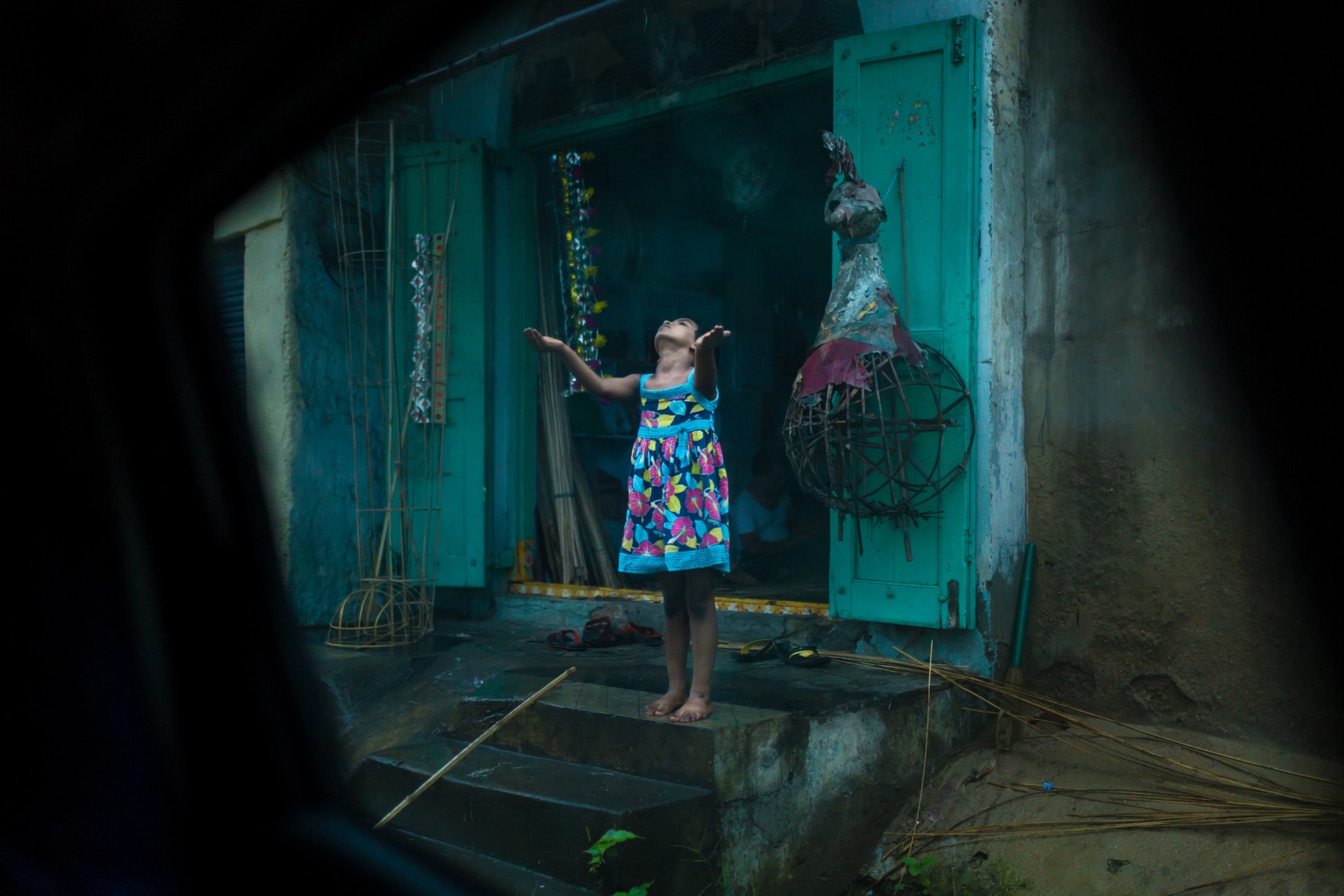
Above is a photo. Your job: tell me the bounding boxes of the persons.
[525,316,733,723]
